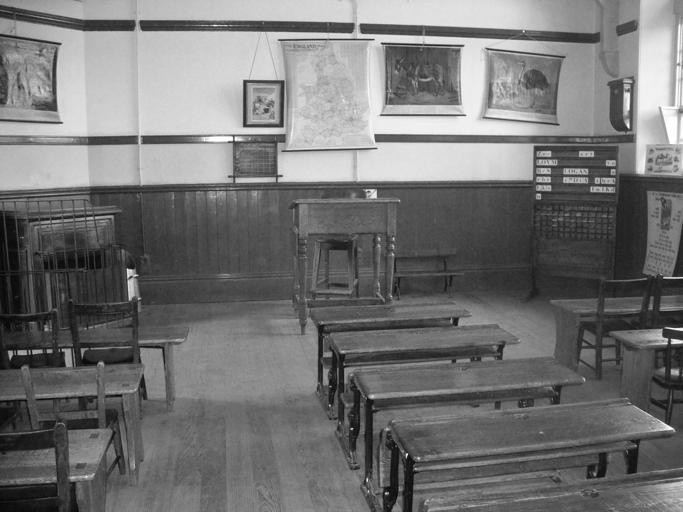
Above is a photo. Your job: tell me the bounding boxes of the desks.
[0,326,189,411]
[287,197,401,335]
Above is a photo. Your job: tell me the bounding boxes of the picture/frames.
[242,79,284,128]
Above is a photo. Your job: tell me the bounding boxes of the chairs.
[307,188,367,298]
[575,273,683,379]
[393,247,464,300]
[0,296,148,503]
